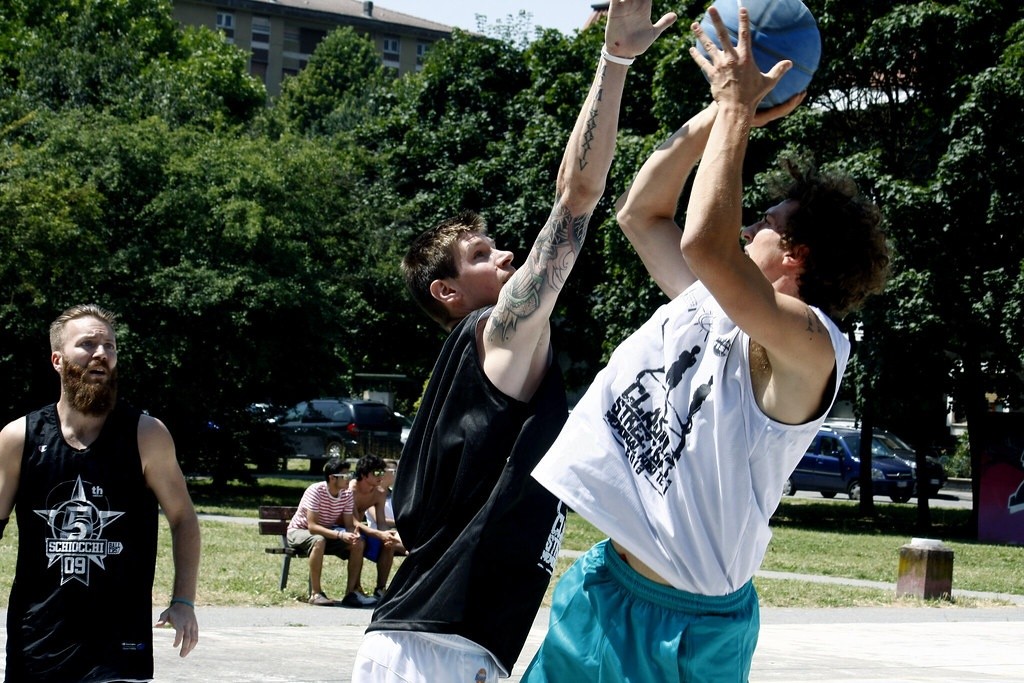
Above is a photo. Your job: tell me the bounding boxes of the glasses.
[332,472,355,481]
[367,470,386,477]
[386,468,397,474]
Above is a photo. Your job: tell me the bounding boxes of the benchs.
[258,505,408,594]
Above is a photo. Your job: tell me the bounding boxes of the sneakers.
[308,591,336,605]
[374,586,387,600]
[341,587,378,607]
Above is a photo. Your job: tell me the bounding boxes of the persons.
[364,457,415,555]
[345,455,401,603]
[272,457,377,606]
[2,302,201,683]
[507,5,886,683]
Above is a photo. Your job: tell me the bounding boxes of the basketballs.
[696,0,819,110]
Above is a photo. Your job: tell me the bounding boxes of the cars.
[860,430,948,497]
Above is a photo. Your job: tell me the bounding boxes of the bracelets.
[336,530,339,539]
[170,597,196,608]
[340,0,681,683]
[340,530,344,539]
[599,45,637,66]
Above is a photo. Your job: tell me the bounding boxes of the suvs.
[268,395,403,459]
[783,423,917,504]
